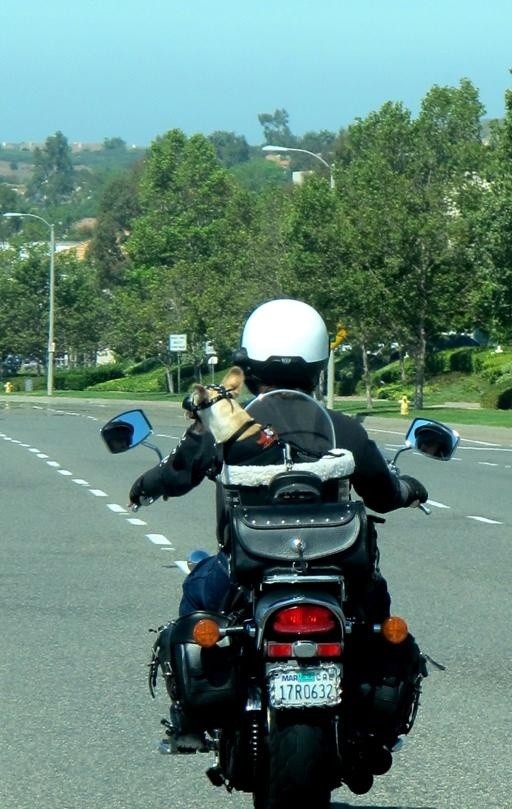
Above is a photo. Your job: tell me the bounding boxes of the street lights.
[262,144,335,410]
[3,211,56,398]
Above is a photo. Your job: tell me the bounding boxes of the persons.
[412,424,452,457]
[102,421,133,451]
[128,296,419,751]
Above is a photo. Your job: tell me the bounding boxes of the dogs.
[181,366,313,467]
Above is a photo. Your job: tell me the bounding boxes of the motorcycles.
[103,385,462,809]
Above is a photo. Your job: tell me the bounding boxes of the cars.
[391,333,478,361]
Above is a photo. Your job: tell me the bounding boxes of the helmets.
[241,299,330,370]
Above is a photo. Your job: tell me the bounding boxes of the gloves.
[397,475,428,506]
[129,476,148,506]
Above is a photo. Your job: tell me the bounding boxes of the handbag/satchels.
[230,502,368,585]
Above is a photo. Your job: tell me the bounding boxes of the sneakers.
[170,705,205,749]
